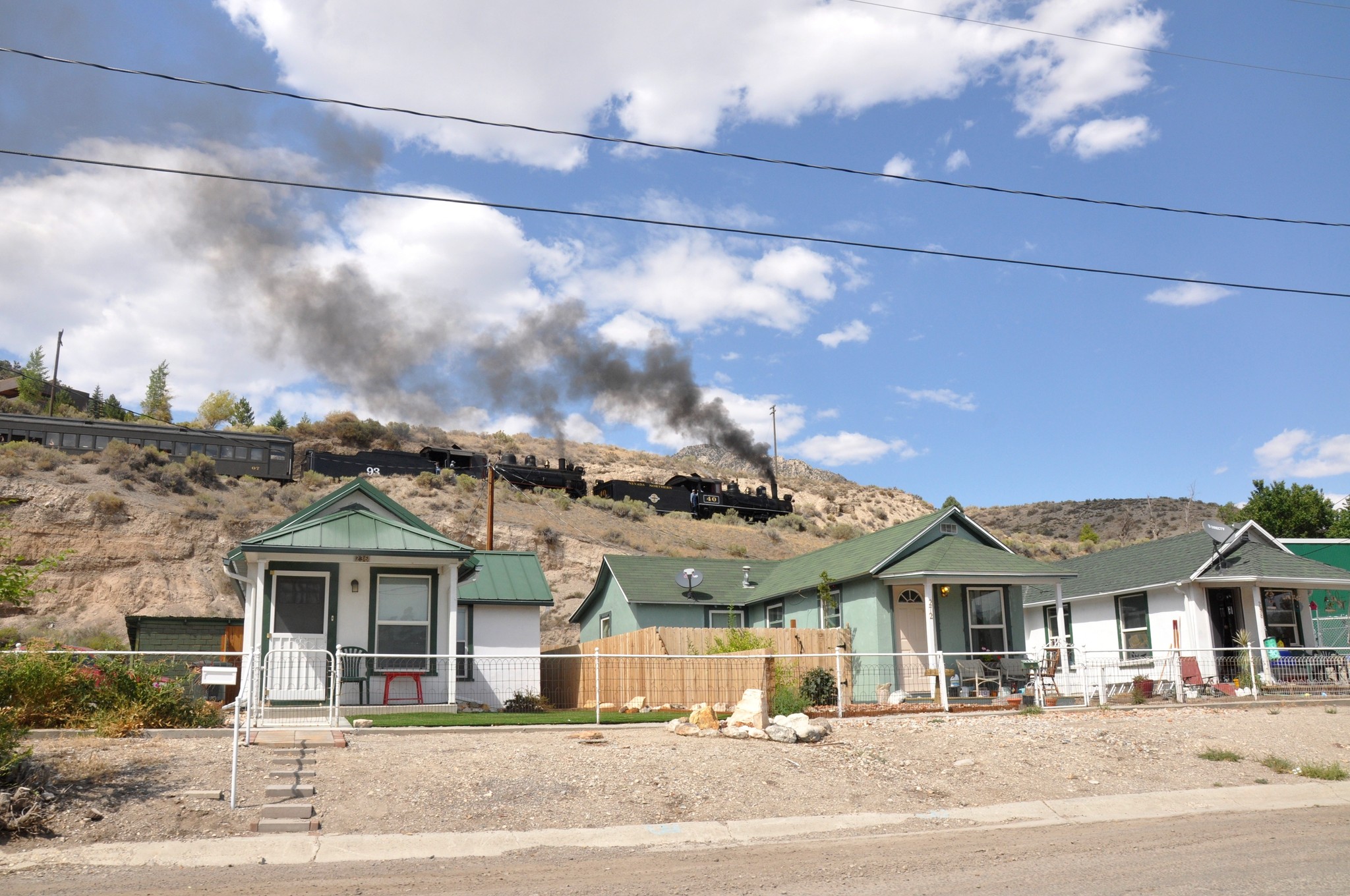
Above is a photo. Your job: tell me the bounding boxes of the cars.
[0,642,181,712]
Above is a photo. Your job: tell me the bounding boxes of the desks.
[382,672,426,706]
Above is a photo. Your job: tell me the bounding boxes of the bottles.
[1277,639,1284,647]
[950,673,960,687]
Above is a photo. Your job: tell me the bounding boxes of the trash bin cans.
[192,660,233,703]
[1264,636,1281,660]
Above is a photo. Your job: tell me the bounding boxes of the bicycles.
[1167,675,1223,697]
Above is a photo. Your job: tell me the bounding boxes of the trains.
[0,413,796,525]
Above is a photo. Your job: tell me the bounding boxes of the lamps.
[938,583,951,598]
[351,579,359,593]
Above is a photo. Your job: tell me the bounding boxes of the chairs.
[1026,648,1062,697]
[1312,649,1350,685]
[999,657,1036,694]
[1269,651,1297,682]
[1288,650,1328,685]
[334,646,374,705]
[955,659,1002,697]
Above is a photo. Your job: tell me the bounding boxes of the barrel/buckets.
[997,682,1012,697]
